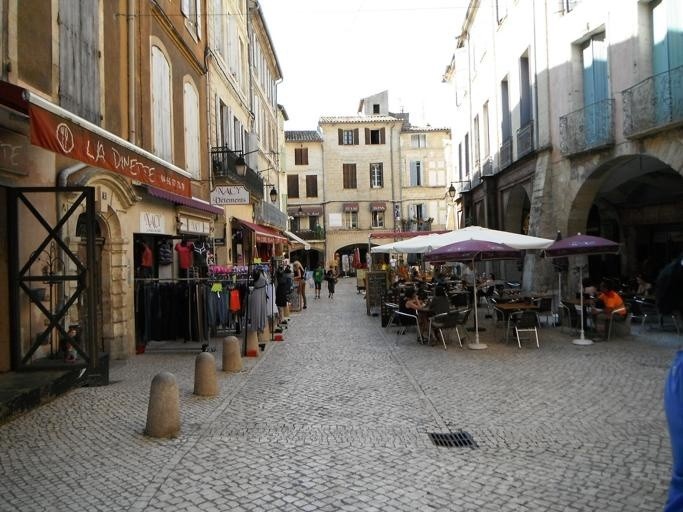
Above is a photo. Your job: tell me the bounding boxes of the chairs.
[384,263,683,350]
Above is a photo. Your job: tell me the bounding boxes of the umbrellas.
[371,232,440,253]
[541,230,623,330]
[351,248,362,269]
[389,224,554,340]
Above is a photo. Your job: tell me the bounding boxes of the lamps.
[449,180,473,198]
[234,150,247,178]
[268,183,279,206]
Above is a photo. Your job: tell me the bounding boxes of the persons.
[398,256,404,264]
[426,284,450,347]
[476,272,494,308]
[389,255,396,268]
[461,264,480,306]
[293,260,306,309]
[411,271,426,282]
[632,273,652,295]
[252,264,266,332]
[272,265,288,323]
[310,263,321,299]
[589,278,626,340]
[325,265,336,298]
[398,287,427,342]
[264,273,283,333]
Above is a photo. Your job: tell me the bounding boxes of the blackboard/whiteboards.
[365,271,388,306]
